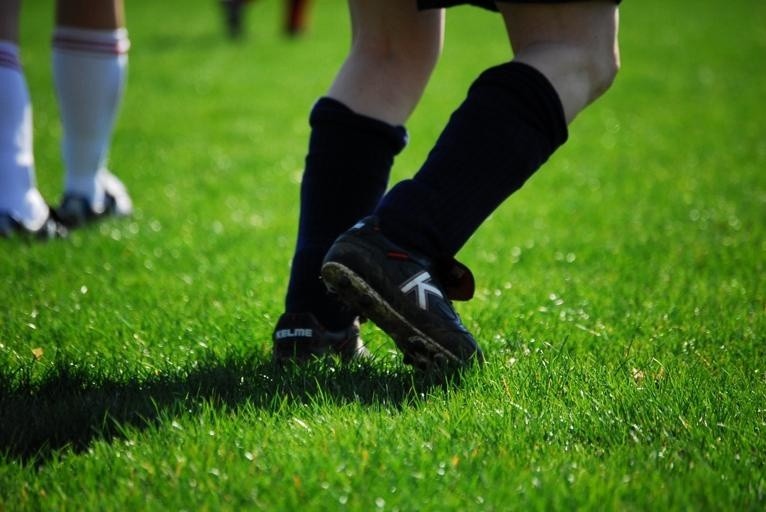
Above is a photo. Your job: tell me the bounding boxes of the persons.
[219,0,317,43]
[269,1,626,393]
[0,0,135,249]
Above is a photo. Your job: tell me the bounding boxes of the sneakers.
[322,214,485,384]
[1,205,77,237]
[272,311,371,361]
[60,185,115,226]
